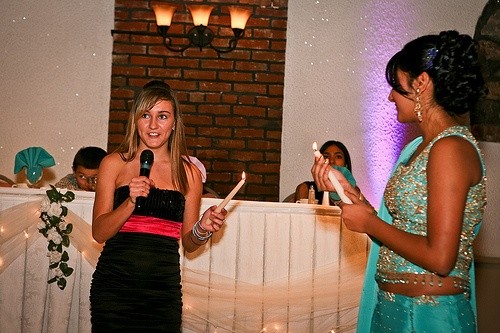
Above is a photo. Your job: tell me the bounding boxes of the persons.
[296,139,361,204]
[311,29,487,333]
[89,80,227,333]
[55,146,105,191]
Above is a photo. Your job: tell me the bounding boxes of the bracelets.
[190,214,212,246]
[359,193,364,202]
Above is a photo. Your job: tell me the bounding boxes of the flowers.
[37,184,74,290]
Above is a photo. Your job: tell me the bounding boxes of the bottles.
[308,185,316,204]
[322,191,329,206]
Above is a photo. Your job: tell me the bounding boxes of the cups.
[296,199,319,204]
[12,183,27,188]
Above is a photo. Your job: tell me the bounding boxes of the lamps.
[149,2,253,52]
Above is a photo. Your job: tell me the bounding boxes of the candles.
[206,171,246,225]
[312,142,354,204]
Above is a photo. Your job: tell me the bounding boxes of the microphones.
[135,150,154,207]
